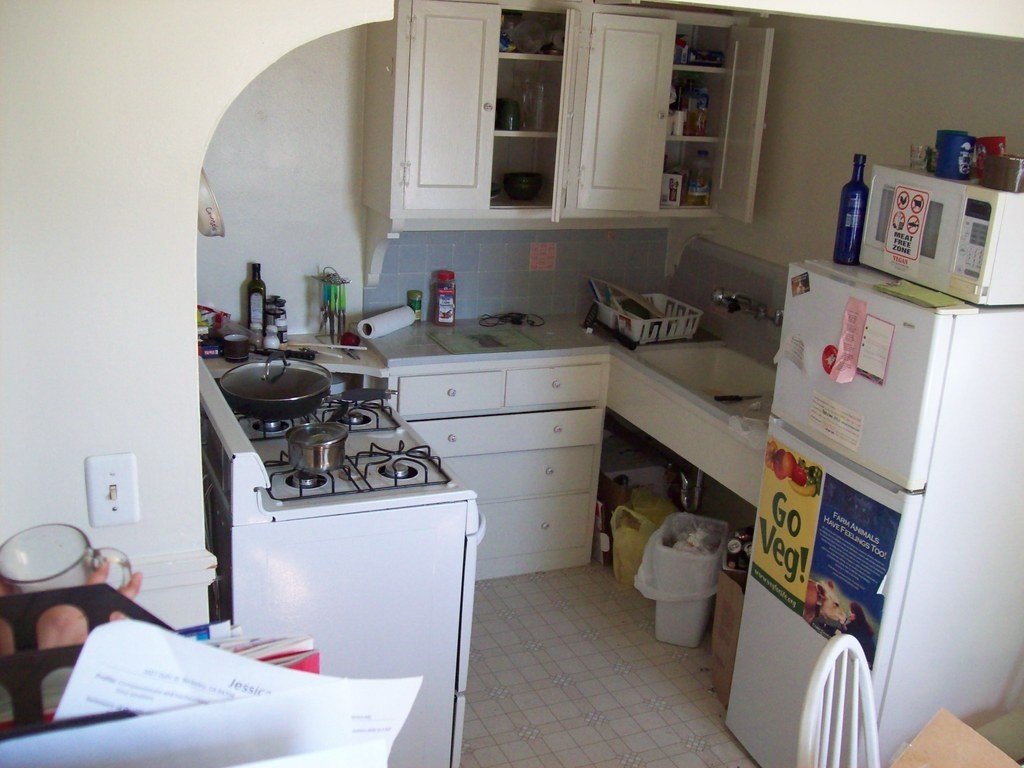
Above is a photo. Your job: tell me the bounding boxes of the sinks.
[634,347,776,407]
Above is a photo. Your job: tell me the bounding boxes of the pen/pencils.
[299,348,342,358]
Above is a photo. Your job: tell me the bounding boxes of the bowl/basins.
[504,172,544,200]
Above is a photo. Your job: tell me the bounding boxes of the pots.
[220,351,333,420]
[285,401,349,473]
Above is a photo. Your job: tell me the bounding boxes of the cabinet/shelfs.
[362,0,779,287]
[387,354,611,581]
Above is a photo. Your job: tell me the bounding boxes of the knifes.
[322,282,346,345]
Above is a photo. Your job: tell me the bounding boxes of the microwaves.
[858,164,1024,305]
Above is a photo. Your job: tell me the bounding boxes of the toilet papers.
[356,305,416,339]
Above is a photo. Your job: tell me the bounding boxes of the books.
[0,618,320,731]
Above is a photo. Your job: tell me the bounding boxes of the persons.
[841,602,877,671]
[0,561,143,655]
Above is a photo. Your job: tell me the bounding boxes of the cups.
[223,334,249,363]
[494,98,522,131]
[522,81,558,131]
[980,155,1024,193]
[934,134,976,180]
[976,136,1006,178]
[910,145,928,169]
[0,523,132,596]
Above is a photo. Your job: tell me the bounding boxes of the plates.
[491,183,500,200]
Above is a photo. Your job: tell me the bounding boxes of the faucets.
[709,287,767,321]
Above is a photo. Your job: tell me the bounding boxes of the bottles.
[434,270,456,327]
[247,263,287,349]
[685,150,712,206]
[833,153,869,265]
[408,290,422,321]
[673,102,684,136]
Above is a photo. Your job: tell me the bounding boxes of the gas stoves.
[198,355,477,526]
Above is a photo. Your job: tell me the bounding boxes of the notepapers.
[876,279,960,307]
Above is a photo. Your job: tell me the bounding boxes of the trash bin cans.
[655,512,729,648]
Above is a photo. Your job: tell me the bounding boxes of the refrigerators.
[725,260,1024,768]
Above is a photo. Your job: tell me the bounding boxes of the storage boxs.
[601,464,669,513]
[198,343,223,359]
[660,169,690,206]
[673,33,724,68]
